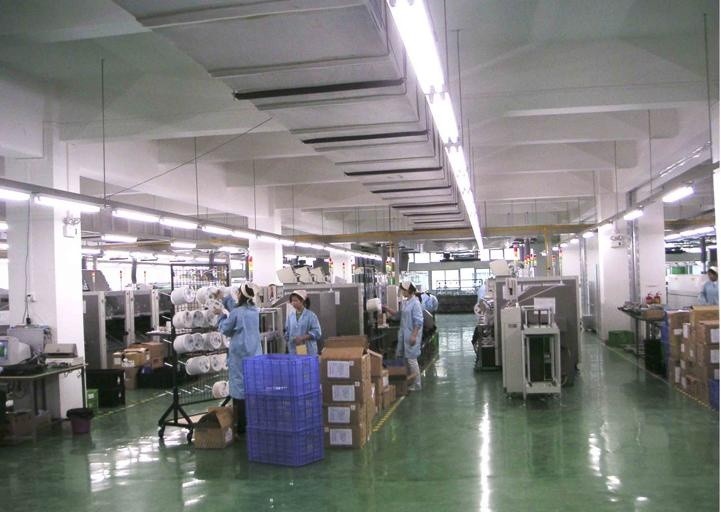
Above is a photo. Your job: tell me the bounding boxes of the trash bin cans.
[644,339,661,373]
[66,407,93,434]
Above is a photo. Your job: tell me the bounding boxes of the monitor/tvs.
[0,336,31,366]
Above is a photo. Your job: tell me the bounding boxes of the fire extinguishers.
[646,292,661,304]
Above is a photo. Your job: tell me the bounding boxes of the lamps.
[537,101,699,258]
[0,60,160,261]
[281,186,398,264]
[161,137,279,255]
[387,0,486,202]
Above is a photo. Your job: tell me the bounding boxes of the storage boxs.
[317,336,407,449]
[0,412,32,441]
[665,305,720,408]
[114,338,165,389]
[194,404,234,449]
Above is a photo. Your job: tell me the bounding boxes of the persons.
[419,289,438,322]
[698,266,718,306]
[212,283,263,439]
[382,280,424,392]
[284,289,322,357]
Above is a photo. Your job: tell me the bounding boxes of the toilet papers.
[173,284,235,399]
[367,297,382,313]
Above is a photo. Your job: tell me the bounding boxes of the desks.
[0,363,88,425]
[617,308,665,359]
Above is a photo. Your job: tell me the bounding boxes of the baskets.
[242,352,323,468]
